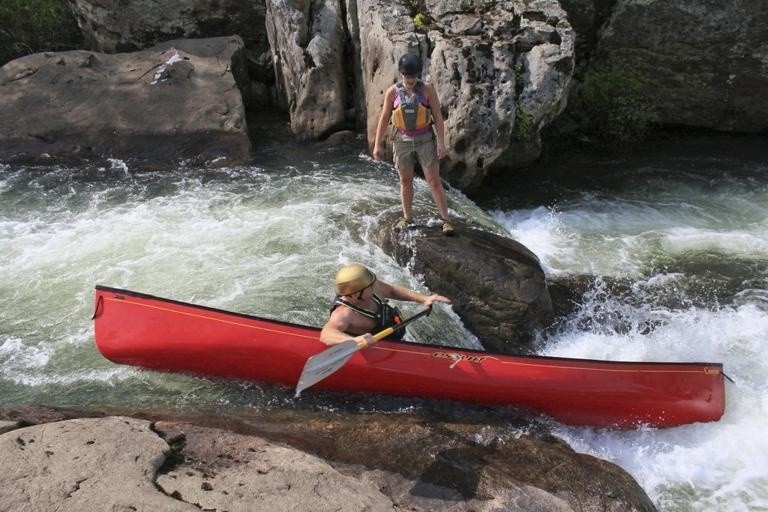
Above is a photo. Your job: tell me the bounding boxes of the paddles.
[295,303,433,392]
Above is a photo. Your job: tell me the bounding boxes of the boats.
[90,284,726,430]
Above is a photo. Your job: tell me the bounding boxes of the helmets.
[335,264,376,296]
[398,54,420,74]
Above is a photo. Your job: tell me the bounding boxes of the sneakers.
[441,220,454,234]
[394,219,411,233]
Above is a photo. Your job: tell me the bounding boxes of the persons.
[319,262,452,348]
[371,54,457,237]
